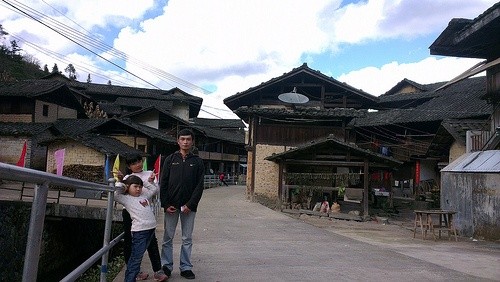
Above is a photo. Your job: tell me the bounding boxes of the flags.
[105,160,109,181]
[16,143,27,166]
[154,154,161,181]
[113,154,119,181]
[142,159,147,171]
[54,149,65,176]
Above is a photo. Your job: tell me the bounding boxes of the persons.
[114,175,158,282]
[219,171,230,187]
[160,129,205,279]
[110,151,160,282]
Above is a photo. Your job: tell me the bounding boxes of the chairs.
[426,178,440,197]
[419,180,432,199]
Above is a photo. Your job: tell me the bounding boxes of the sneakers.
[163,266,171,277]
[181,270,195,279]
[153,270,168,282]
[136,271,149,280]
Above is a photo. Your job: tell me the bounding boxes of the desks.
[410,210,458,241]
